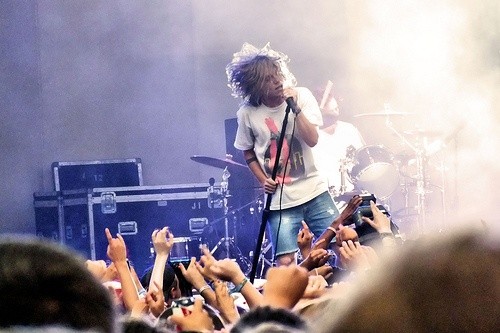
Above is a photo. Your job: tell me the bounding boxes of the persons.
[313,89,372,213]
[0,194,500,333]
[226,45,339,264]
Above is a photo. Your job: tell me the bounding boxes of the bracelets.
[237,277,249,292]
[293,107,302,116]
[379,233,393,240]
[199,285,211,293]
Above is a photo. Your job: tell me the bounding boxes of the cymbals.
[189,155,255,177]
[352,109,417,118]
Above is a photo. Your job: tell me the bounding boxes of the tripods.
[212,191,273,278]
[210,167,249,273]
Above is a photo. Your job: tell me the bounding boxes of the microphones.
[282,89,301,114]
[209,177,215,207]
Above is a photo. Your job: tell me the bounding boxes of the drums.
[351,218,402,248]
[349,143,401,202]
[149,236,209,264]
